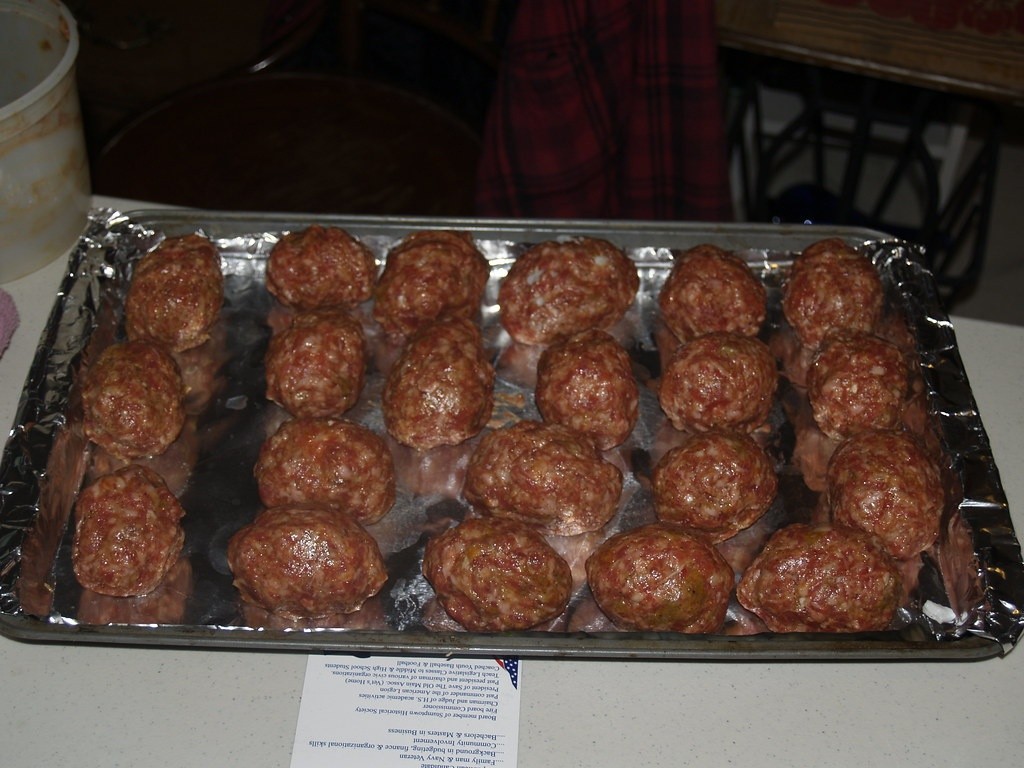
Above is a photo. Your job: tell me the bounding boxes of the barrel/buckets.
[0,0,91,287]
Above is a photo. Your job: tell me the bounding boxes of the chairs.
[91,0,509,217]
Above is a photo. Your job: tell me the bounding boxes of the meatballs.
[71,226,946,639]
